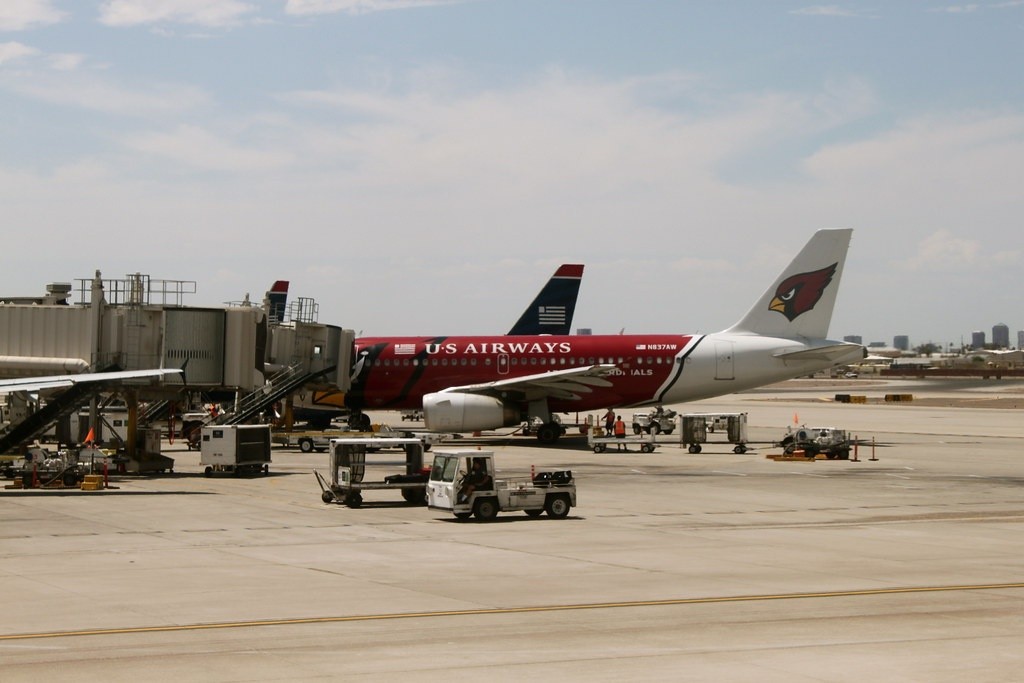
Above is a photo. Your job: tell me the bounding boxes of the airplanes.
[277,228,868,446]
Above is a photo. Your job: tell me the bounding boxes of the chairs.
[533,470,573,487]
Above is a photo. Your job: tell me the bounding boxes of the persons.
[657,405,664,416]
[210,403,219,420]
[457,458,489,504]
[601,409,615,437]
[614,416,629,453]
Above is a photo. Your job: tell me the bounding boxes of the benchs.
[464,473,494,506]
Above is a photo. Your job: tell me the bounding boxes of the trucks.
[632,409,676,435]
[426,450,577,523]
